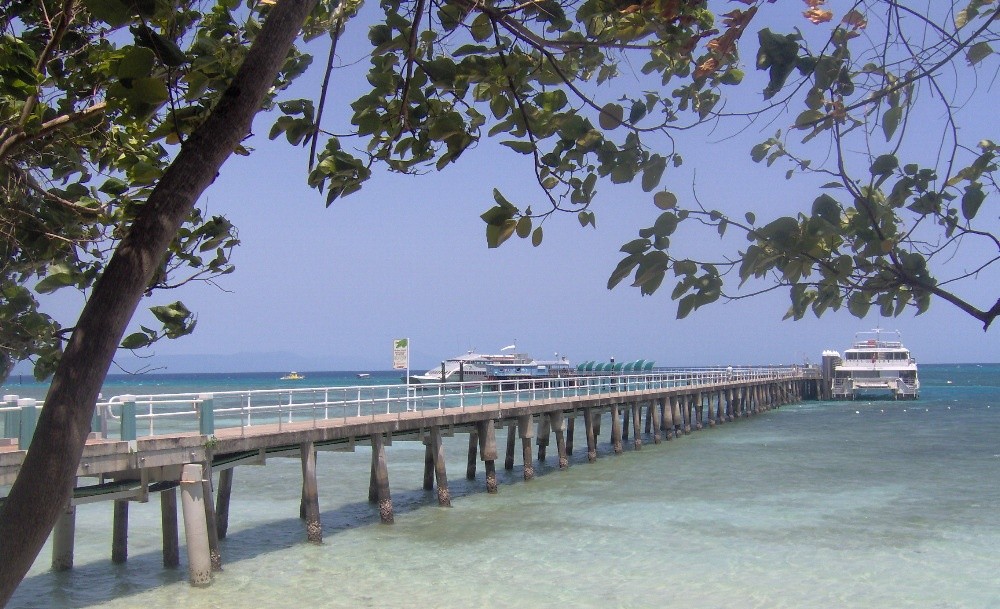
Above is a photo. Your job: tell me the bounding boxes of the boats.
[401,339,578,390]
[356,374,370,379]
[280,371,306,380]
[830,312,920,401]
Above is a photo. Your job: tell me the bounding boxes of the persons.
[727,365,733,384]
